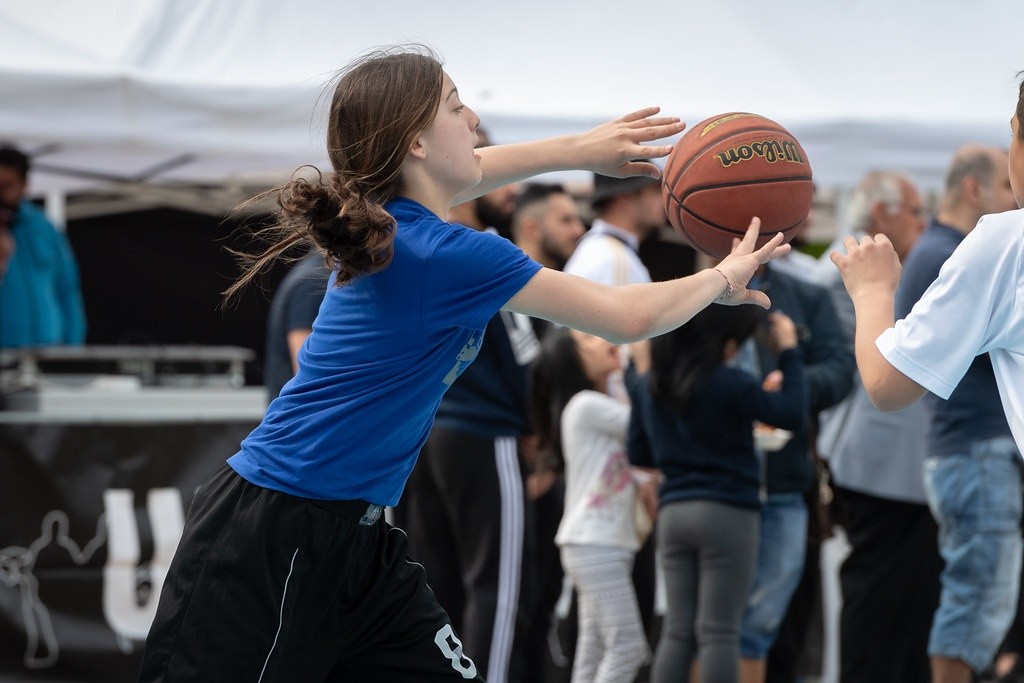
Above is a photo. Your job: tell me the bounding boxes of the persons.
[263,81,1024,682]
[0,146,87,350]
[143,53,793,683]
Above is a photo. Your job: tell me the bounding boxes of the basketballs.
[660,111,816,261]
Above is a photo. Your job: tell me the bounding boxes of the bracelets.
[714,269,733,302]
